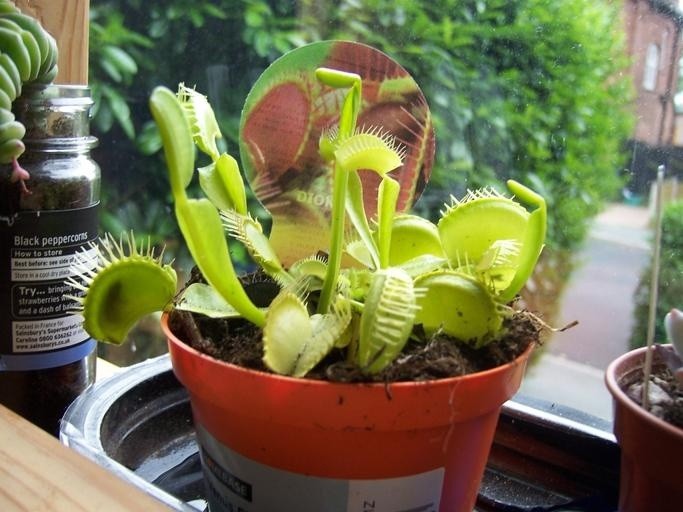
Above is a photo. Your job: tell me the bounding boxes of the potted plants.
[0,0,104,373]
[604,307,683,512]
[62,68,548,512]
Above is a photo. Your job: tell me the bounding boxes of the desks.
[0,406,187,512]
